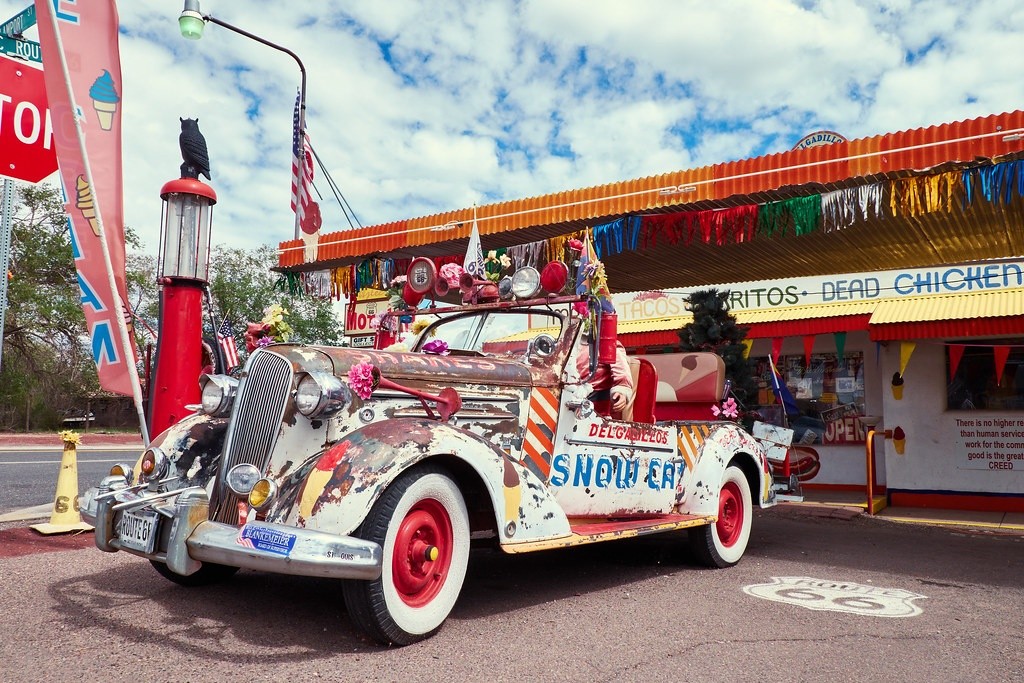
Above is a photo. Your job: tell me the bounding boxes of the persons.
[552,310,634,420]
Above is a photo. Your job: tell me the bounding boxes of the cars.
[81,256,777,647]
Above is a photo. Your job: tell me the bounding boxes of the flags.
[770,361,800,415]
[464,210,489,281]
[577,237,615,313]
[215,314,240,373]
[290,93,323,235]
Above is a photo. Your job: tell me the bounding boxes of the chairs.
[626,357,658,426]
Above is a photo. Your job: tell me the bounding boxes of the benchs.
[632,353,726,424]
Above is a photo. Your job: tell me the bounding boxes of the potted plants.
[859,414,882,428]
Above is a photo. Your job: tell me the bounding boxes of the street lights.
[180,0,308,240]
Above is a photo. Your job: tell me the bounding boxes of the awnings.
[482,299,880,353]
[870,289,1024,343]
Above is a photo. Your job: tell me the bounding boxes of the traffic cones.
[28,433,95,536]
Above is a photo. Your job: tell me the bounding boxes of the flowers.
[346,360,376,401]
[437,261,466,285]
[421,338,451,358]
[384,342,413,352]
[256,308,290,346]
[579,259,612,346]
[59,429,82,446]
[711,394,740,419]
[482,246,506,283]
[386,274,409,311]
